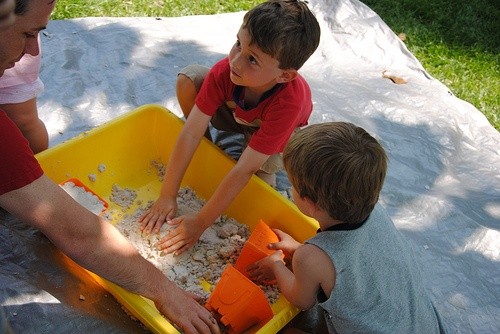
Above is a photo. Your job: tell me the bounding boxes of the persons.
[140,0,320,258]
[245,122,448,334]
[0,0,222,334]
[0,32,49,155]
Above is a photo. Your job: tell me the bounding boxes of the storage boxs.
[34,103,320,334]
